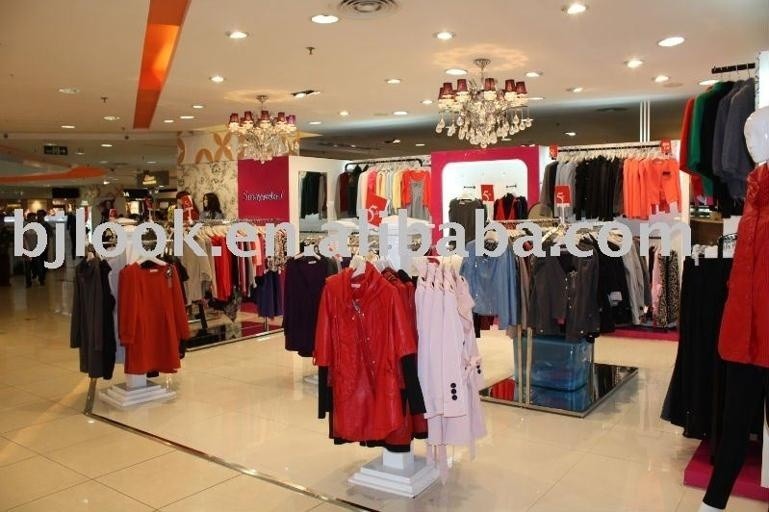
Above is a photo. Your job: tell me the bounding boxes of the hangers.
[548,143,676,164]
[693,64,754,97]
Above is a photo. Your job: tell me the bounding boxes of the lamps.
[227,97,301,165]
[434,58,533,149]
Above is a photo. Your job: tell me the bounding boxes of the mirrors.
[297,170,328,253]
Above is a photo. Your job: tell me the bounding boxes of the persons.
[1,206,166,288]
[700,105,769,510]
[166,191,196,224]
[198,193,226,220]
